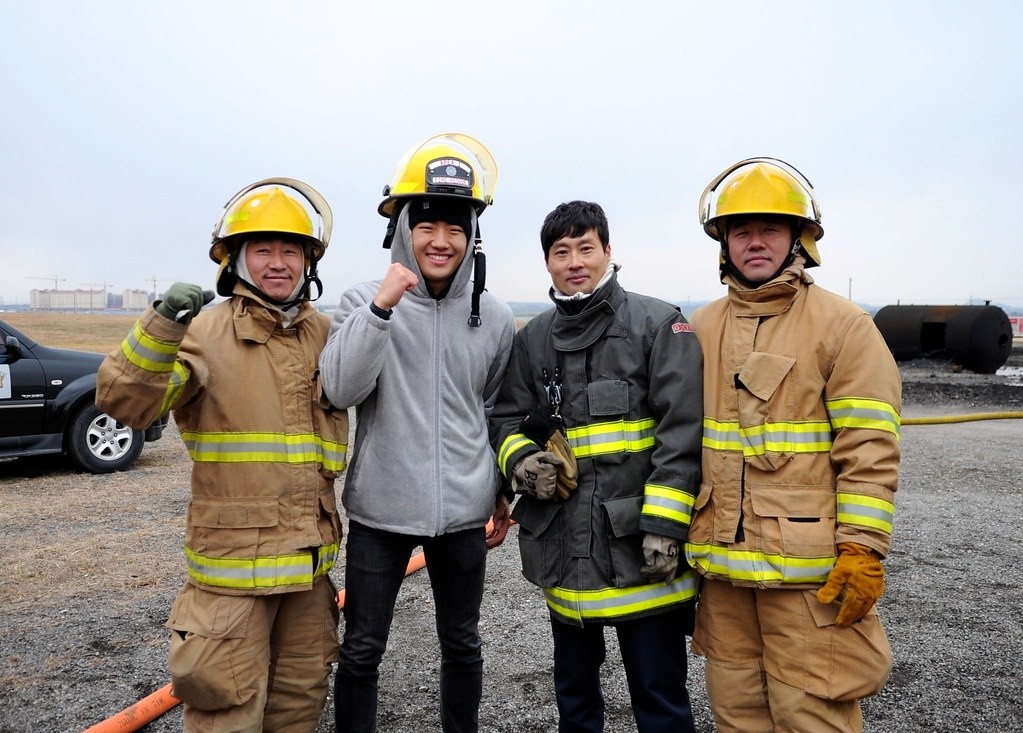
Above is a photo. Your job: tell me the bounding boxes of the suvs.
[0,319,169,475]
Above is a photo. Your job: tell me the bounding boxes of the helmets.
[703,162,824,242]
[377,138,487,219]
[209,183,325,264]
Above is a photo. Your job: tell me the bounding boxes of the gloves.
[156,281,215,325]
[521,406,578,503]
[816,542,884,628]
[511,451,562,500]
[639,532,680,586]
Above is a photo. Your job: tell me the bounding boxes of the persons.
[95,178,351,733]
[684,154,902,733]
[488,201,705,733]
[319,132,518,733]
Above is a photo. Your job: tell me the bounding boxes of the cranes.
[24,274,66,289]
[81,282,114,290]
[144,276,175,290]
